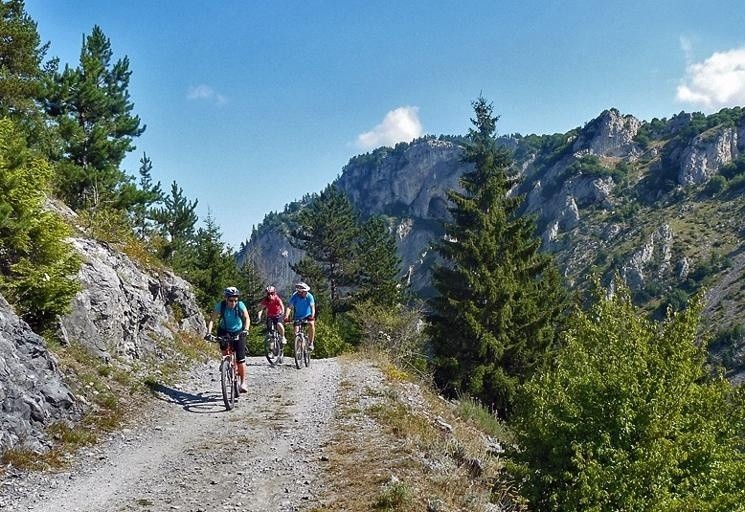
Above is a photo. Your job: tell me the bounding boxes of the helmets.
[295,282,311,291]
[224,287,239,296]
[266,285,277,293]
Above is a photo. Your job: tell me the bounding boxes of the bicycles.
[207,333,246,410]
[255,315,286,366]
[286,319,315,369]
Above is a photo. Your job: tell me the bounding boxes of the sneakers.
[268,350,274,359]
[278,335,287,344]
[240,385,247,393]
[306,343,314,350]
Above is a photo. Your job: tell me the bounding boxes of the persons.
[206,286,250,393]
[283,282,316,350]
[255,285,287,345]
